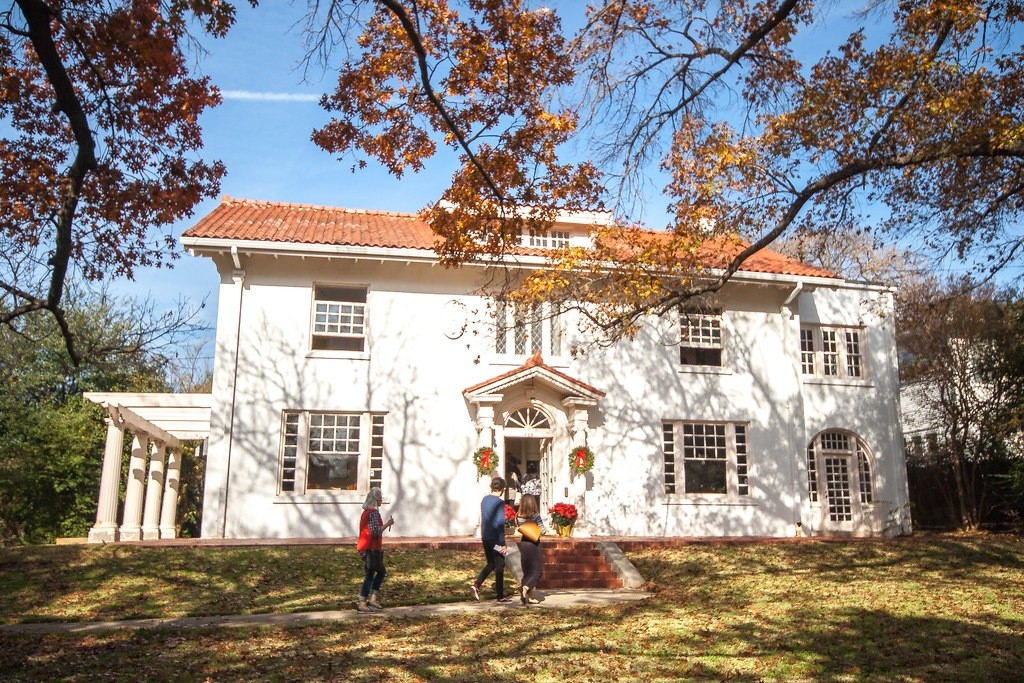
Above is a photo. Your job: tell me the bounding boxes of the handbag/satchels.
[518,517,541,542]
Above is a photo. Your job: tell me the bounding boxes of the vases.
[505,527,515,535]
[481,450,491,470]
[559,524,571,538]
[576,450,587,467]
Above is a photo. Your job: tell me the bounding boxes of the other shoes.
[357,604,376,614]
[518,584,529,604]
[496,597,513,605]
[527,596,539,604]
[470,585,479,601]
[366,600,382,611]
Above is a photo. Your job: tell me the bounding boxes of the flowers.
[472,446,499,480]
[504,505,519,528]
[569,446,595,473]
[547,503,579,526]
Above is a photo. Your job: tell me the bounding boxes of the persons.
[505,452,542,514]
[470,477,514,605]
[356,487,395,614]
[512,493,546,605]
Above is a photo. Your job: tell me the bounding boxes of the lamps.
[530,378,536,403]
